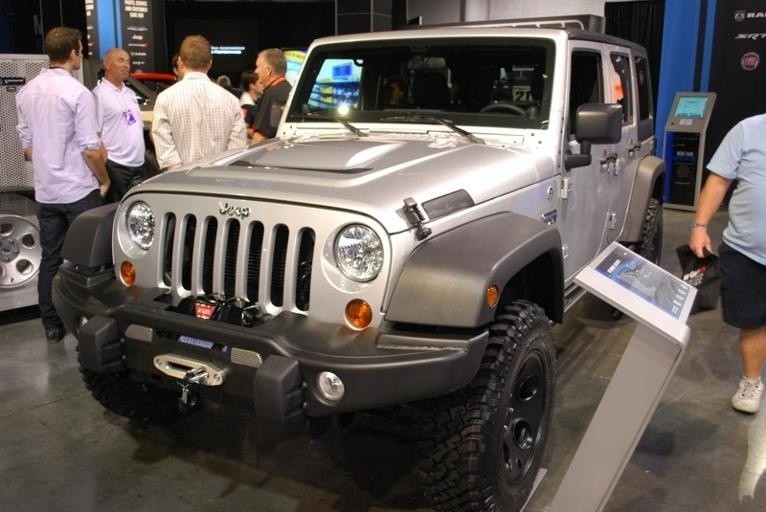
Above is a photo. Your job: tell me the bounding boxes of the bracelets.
[692,223,707,228]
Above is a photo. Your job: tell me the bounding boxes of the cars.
[114,67,181,129]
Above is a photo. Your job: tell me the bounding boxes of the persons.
[216,74,240,100]
[92,48,147,202]
[248,47,293,150]
[237,69,265,139]
[171,53,186,87]
[149,33,247,172]
[14,27,112,345]
[688,114,766,415]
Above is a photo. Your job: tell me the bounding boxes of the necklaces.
[49,65,72,73]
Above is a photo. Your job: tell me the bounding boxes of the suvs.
[50,26,665,503]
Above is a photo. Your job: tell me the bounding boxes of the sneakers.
[45,327,67,342]
[732,377,764,413]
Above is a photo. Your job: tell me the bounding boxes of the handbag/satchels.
[676,245,721,315]
